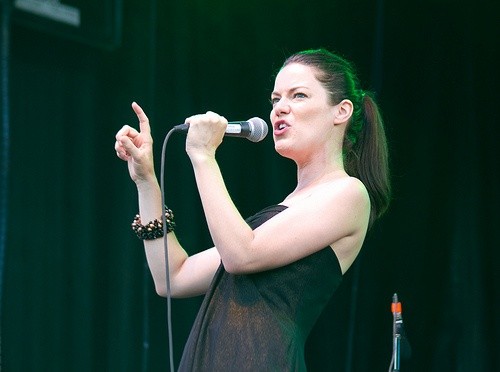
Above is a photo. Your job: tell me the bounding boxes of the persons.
[113,50,390,372]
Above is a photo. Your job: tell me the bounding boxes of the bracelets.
[132,205,175,240]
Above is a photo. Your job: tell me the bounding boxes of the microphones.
[174,116,269,143]
[393,294,402,335]
